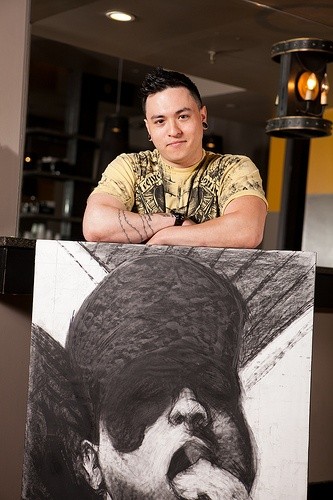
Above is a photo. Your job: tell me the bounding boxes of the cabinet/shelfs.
[19,126,141,224]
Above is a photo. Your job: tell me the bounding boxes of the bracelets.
[172,213,185,226]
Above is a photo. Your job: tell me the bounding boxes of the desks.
[0,237,333,313]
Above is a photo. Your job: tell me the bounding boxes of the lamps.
[296,71,331,117]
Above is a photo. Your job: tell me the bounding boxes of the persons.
[83,68,269,249]
